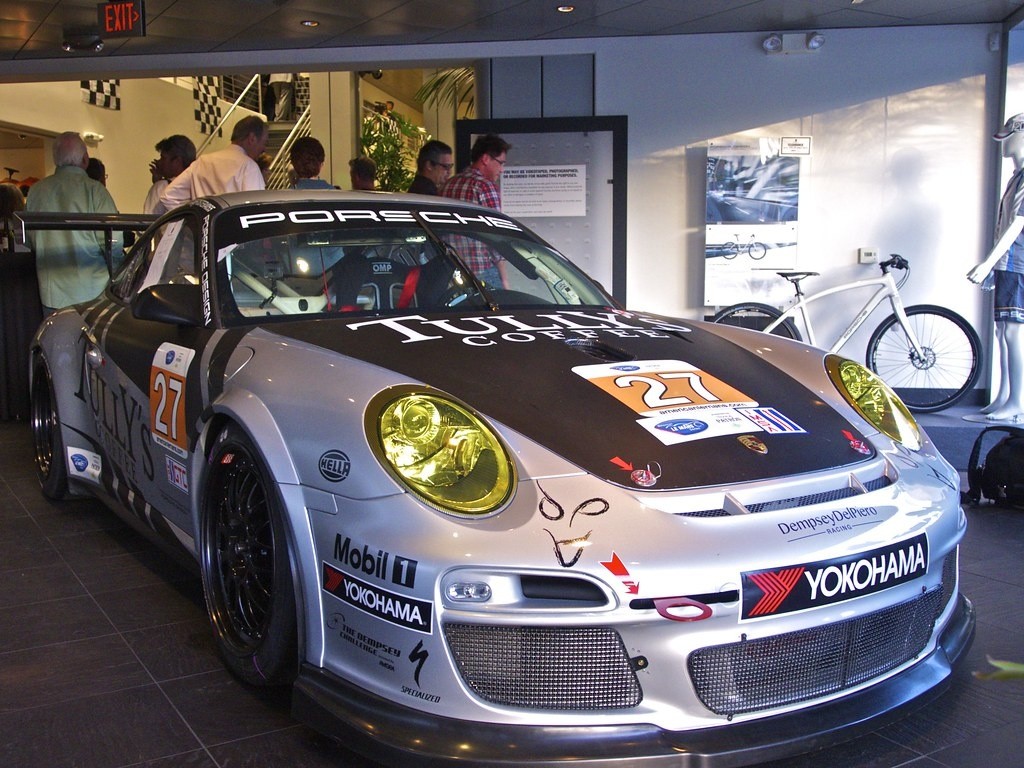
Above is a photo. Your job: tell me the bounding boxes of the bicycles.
[710,253,983,414]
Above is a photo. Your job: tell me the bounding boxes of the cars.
[32,191,974,767]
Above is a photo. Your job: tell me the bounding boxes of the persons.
[442,134,513,291]
[24,131,125,321]
[86,157,107,187]
[160,116,273,285]
[408,141,455,197]
[288,137,344,277]
[1,184,46,424]
[136,134,197,236]
[967,113,1024,420]
[380,101,394,118]
[268,73,293,121]
[252,152,272,187]
[337,158,387,298]
[293,72,310,123]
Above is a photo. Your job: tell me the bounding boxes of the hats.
[992,112,1024,142]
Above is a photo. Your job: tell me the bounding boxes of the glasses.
[440,163,454,170]
[489,154,506,167]
[98,174,108,179]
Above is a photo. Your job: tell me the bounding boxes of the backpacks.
[959,426,1024,511]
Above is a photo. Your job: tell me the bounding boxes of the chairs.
[340,256,436,313]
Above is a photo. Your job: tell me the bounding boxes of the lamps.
[762,31,826,55]
[61,24,104,54]
